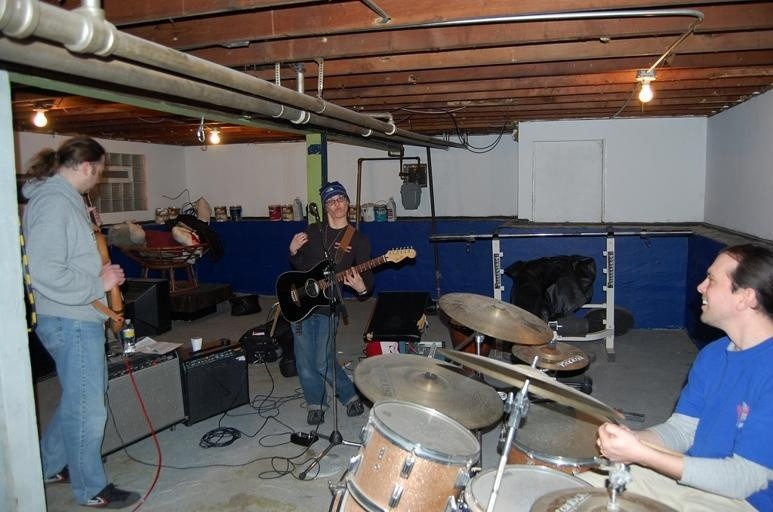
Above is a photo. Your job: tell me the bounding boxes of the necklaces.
[323,224,344,258]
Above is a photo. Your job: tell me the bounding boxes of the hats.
[319,182,350,208]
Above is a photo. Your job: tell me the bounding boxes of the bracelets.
[356,290,368,295]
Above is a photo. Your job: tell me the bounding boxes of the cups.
[191,336,203,351]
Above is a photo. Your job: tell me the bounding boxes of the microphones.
[309,202,319,218]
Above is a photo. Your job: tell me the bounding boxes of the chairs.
[120,228,209,296]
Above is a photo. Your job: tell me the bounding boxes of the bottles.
[122,318,136,356]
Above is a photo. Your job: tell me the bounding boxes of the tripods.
[299,281,365,482]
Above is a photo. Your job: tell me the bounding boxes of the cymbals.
[529,486,678,512]
[355,354,504,430]
[513,341,589,371]
[440,292,553,345]
[437,348,625,419]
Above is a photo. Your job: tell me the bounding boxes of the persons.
[288,182,373,424]
[21,136,142,509]
[574,242,772,512]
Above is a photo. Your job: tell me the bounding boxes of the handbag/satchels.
[229,295,261,316]
[502,255,597,324]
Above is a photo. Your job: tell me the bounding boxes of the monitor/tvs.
[122,277,171,339]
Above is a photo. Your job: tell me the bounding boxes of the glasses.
[325,197,345,205]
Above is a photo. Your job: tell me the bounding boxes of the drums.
[458,465,593,512]
[340,398,480,512]
[498,400,619,474]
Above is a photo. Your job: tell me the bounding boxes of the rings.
[120,272,123,278]
[302,237,306,241]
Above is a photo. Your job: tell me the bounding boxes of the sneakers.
[44,465,71,485]
[347,399,364,416]
[308,410,324,425]
[77,484,141,508]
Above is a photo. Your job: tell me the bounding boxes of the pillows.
[171,221,204,258]
[105,223,147,250]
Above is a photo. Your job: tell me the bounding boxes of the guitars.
[276,246,416,323]
[80,191,124,333]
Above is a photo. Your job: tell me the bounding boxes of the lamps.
[208,127,222,145]
[30,105,49,128]
[633,69,657,104]
